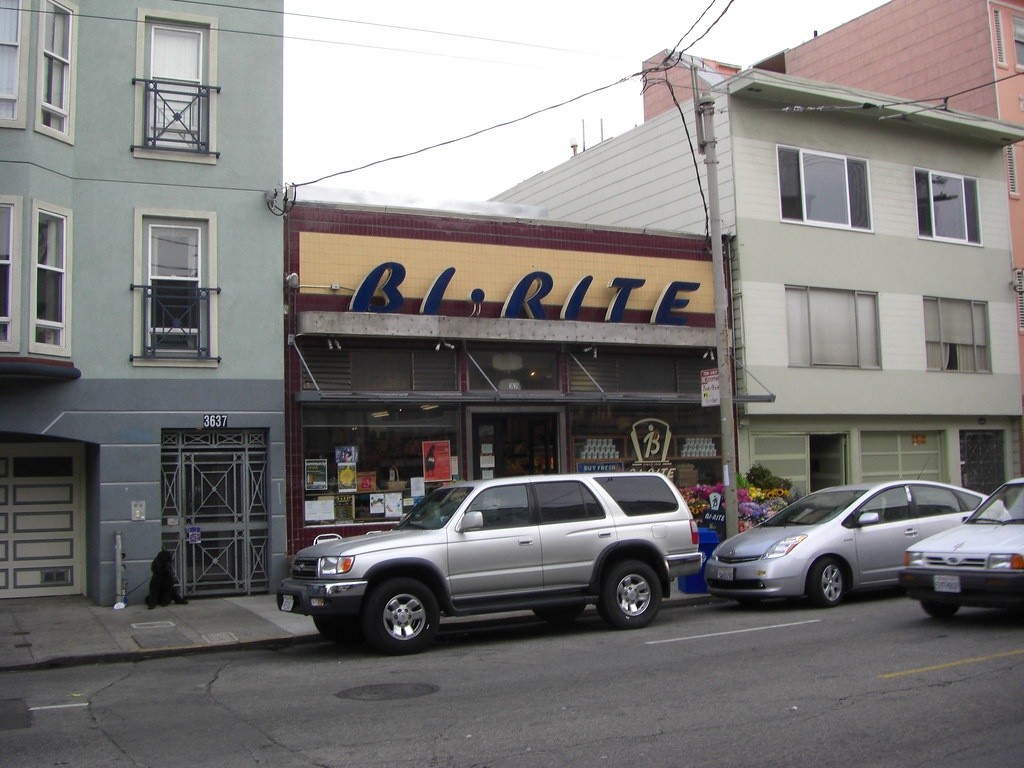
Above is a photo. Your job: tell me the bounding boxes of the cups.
[680,437,716,457]
[581,439,619,459]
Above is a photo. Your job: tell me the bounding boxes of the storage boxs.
[356,471,377,490]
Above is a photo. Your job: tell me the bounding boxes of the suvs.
[276,473,703,654]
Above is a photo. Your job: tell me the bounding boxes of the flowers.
[680,461,806,534]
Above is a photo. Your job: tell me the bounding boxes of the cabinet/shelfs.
[572,434,723,490]
[306,486,441,524]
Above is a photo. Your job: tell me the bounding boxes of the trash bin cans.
[678,527,721,594]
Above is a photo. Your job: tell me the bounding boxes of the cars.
[705,481,1012,608]
[899,477,1024,618]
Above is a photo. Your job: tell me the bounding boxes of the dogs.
[144,550,188,610]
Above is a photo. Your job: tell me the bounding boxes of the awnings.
[296,379,776,406]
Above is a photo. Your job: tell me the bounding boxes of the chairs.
[884,492,907,520]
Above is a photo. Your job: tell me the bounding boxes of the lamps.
[703,347,709,359]
[443,337,455,349]
[710,349,715,360]
[583,343,592,353]
[593,343,597,358]
[434,337,441,351]
[334,334,342,350]
[326,334,333,350]
